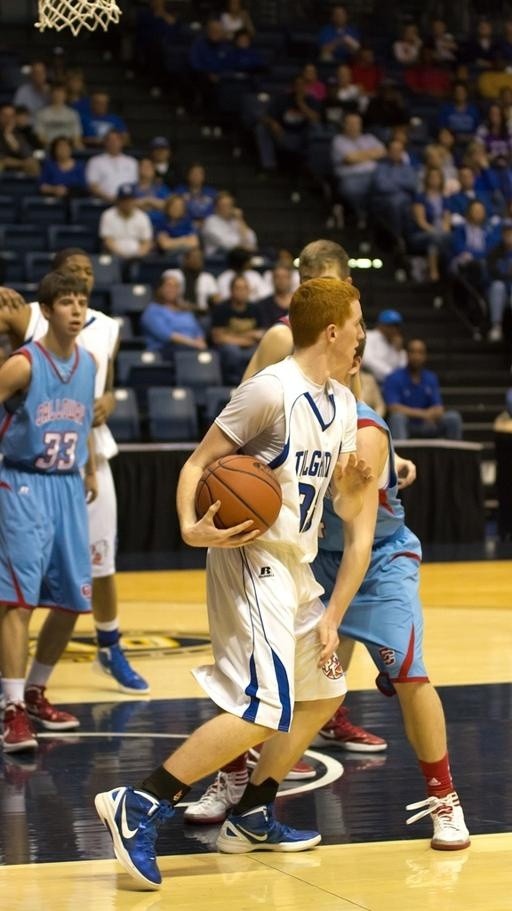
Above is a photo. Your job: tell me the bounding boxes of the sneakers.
[217,809,322,854]
[310,710,388,752]
[185,775,250,822]
[1,699,38,752]
[247,744,315,779]
[95,787,174,888]
[22,684,78,730]
[406,792,470,849]
[97,643,149,691]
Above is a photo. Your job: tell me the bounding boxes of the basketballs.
[196,454,284,538]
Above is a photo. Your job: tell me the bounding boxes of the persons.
[149,135,180,192]
[79,92,129,147]
[388,103,512,343]
[471,20,511,93]
[14,105,36,150]
[40,135,86,196]
[184,311,473,853]
[0,102,41,177]
[143,2,281,158]
[317,8,374,86]
[1,247,154,693]
[428,17,470,86]
[362,310,408,384]
[266,250,300,292]
[177,163,216,221]
[287,64,329,132]
[393,24,448,98]
[131,158,170,229]
[259,266,294,323]
[92,277,375,890]
[442,86,480,148]
[34,81,84,151]
[136,275,207,358]
[328,114,386,199]
[380,338,462,440]
[243,239,388,781]
[62,68,92,133]
[210,276,270,387]
[15,62,51,112]
[201,190,259,267]
[0,270,98,753]
[85,131,139,202]
[327,66,370,122]
[99,183,156,284]
[153,193,204,270]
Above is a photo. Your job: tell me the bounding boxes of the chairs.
[129,364,178,411]
[67,198,108,227]
[110,316,147,349]
[5,281,40,302]
[0,224,43,252]
[0,196,18,221]
[205,388,238,421]
[148,387,198,441]
[0,166,40,196]
[88,287,114,316]
[47,224,90,250]
[0,252,19,280]
[110,284,153,313]
[175,350,222,406]
[117,350,176,385]
[22,197,61,222]
[106,388,139,442]
[89,254,121,289]
[24,252,56,281]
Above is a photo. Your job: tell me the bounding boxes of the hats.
[378,310,401,325]
[117,185,136,203]
[150,135,170,150]
